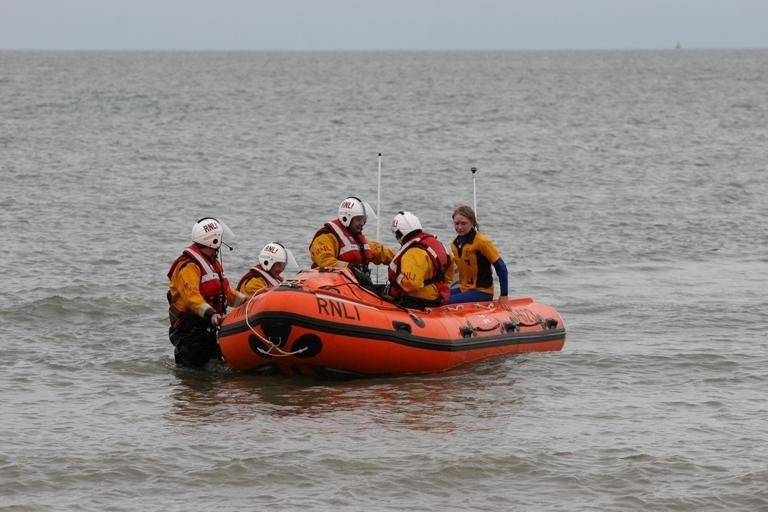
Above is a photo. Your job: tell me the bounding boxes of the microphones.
[221,240,234,252]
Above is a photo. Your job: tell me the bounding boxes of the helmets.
[259,242,299,271]
[337,197,377,228]
[392,212,423,246]
[192,217,235,249]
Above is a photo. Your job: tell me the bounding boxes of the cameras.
[348,260,371,286]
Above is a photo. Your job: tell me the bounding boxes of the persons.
[448,205,512,312]
[166,217,248,371]
[382,212,455,310]
[309,197,395,287]
[236,241,288,297]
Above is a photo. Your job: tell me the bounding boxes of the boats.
[216,265,568,380]
